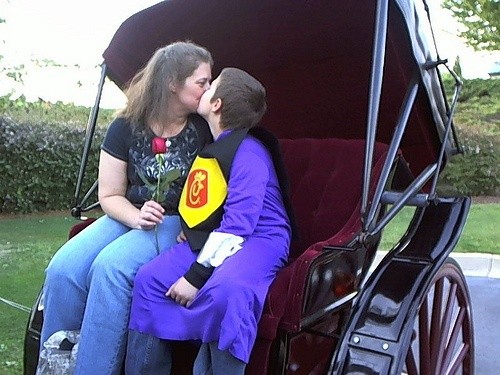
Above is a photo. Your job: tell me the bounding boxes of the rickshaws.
[27,0,475,373]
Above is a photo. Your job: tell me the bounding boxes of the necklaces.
[158,123,173,147]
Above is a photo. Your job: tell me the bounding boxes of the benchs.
[68,137,394,317]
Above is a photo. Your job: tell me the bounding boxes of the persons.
[124,68,293,375]
[35,41,215,375]
